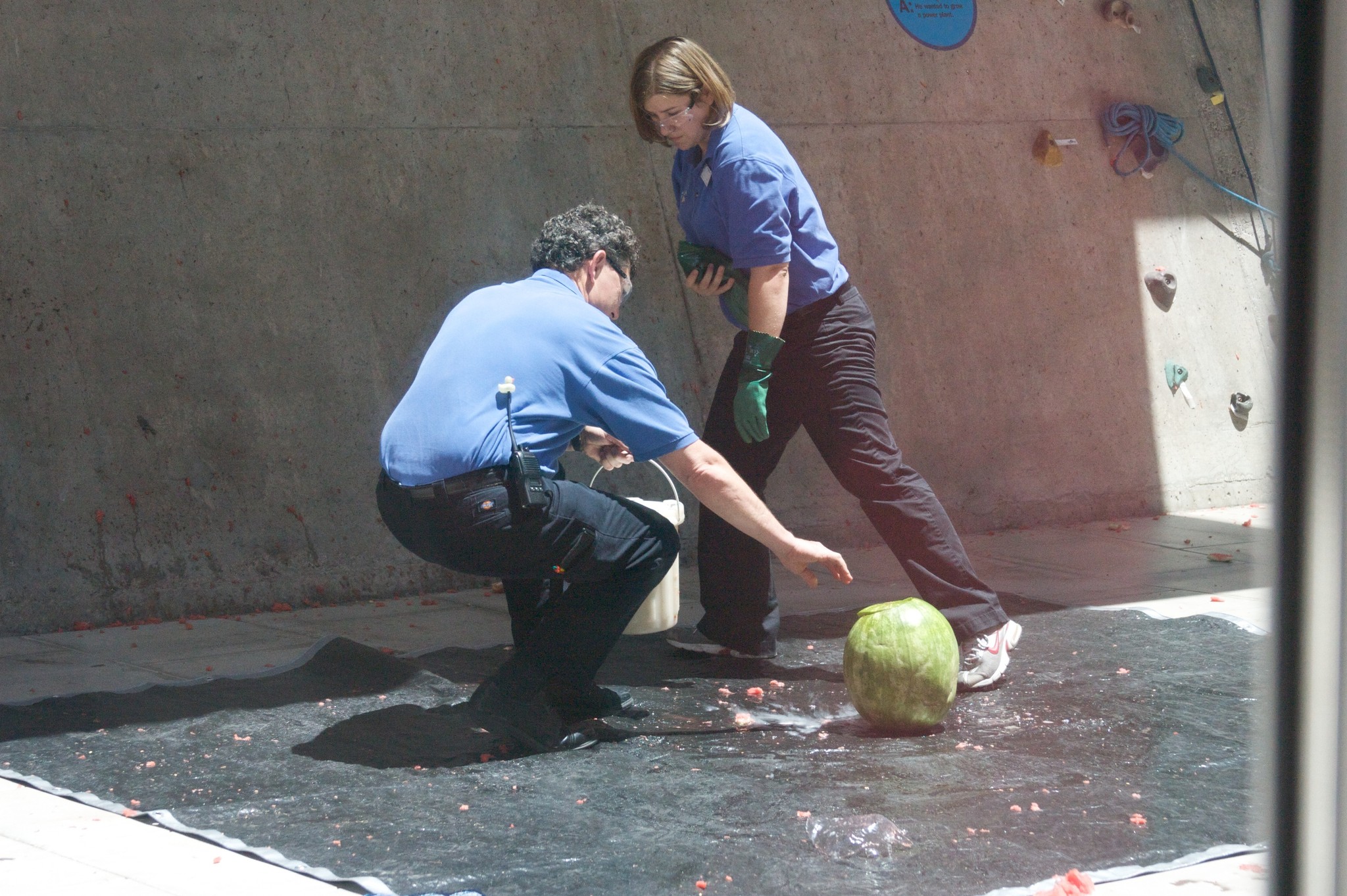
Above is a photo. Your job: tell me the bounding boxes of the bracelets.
[570,433,584,452]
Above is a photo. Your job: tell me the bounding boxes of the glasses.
[606,256,633,303]
[650,91,693,130]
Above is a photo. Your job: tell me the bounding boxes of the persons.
[633,37,1021,692]
[377,206,854,747]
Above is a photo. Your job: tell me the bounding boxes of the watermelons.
[842,596,959,733]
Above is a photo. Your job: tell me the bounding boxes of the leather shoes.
[468,679,598,751]
[540,686,634,718]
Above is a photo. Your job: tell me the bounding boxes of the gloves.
[677,241,750,327]
[733,329,785,444]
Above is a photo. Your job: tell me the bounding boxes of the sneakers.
[666,627,777,659]
[957,619,1023,688]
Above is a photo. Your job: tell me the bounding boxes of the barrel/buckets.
[590,455,686,636]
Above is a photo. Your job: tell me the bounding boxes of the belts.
[381,466,503,499]
